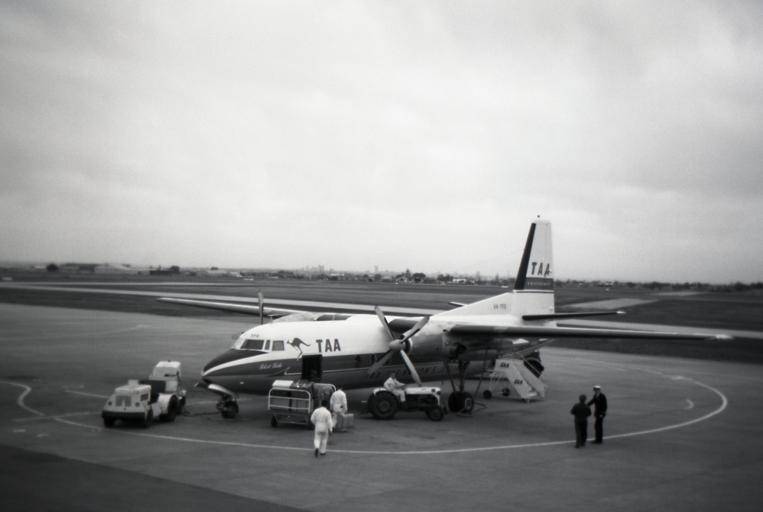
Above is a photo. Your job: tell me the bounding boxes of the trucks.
[267,379,445,430]
[102,359,187,430]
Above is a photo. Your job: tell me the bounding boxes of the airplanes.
[157,215,763,418]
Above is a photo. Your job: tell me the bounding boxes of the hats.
[592,386,601,391]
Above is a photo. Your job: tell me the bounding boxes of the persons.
[587,385,607,443]
[570,395,591,448]
[328,383,348,430]
[384,370,407,404]
[310,400,335,458]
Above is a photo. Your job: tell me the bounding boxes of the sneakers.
[575,438,601,447]
[313,448,325,456]
